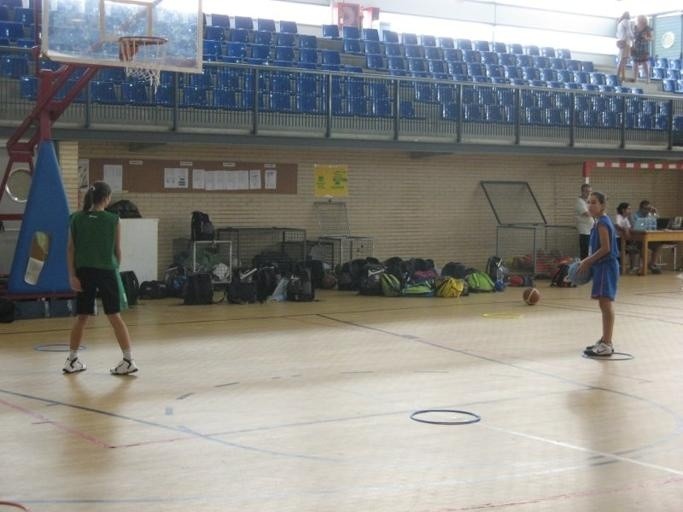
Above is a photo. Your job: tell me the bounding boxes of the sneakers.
[110,358,138,374]
[584,340,614,355]
[62,358,86,373]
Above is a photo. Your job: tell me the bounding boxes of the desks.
[621,228,682,275]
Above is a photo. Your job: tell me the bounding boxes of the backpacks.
[120,257,505,302]
[513,249,573,287]
[191,209,214,240]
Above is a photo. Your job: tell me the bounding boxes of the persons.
[575,190,619,356]
[575,184,597,260]
[633,200,661,274]
[614,202,641,275]
[63,181,138,375]
[631,17,653,81]
[615,11,631,79]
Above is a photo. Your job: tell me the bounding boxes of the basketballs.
[522,288,540,305]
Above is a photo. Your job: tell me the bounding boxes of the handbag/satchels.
[108,200,142,218]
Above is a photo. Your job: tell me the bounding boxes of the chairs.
[0,0,680,129]
[653,242,677,273]
